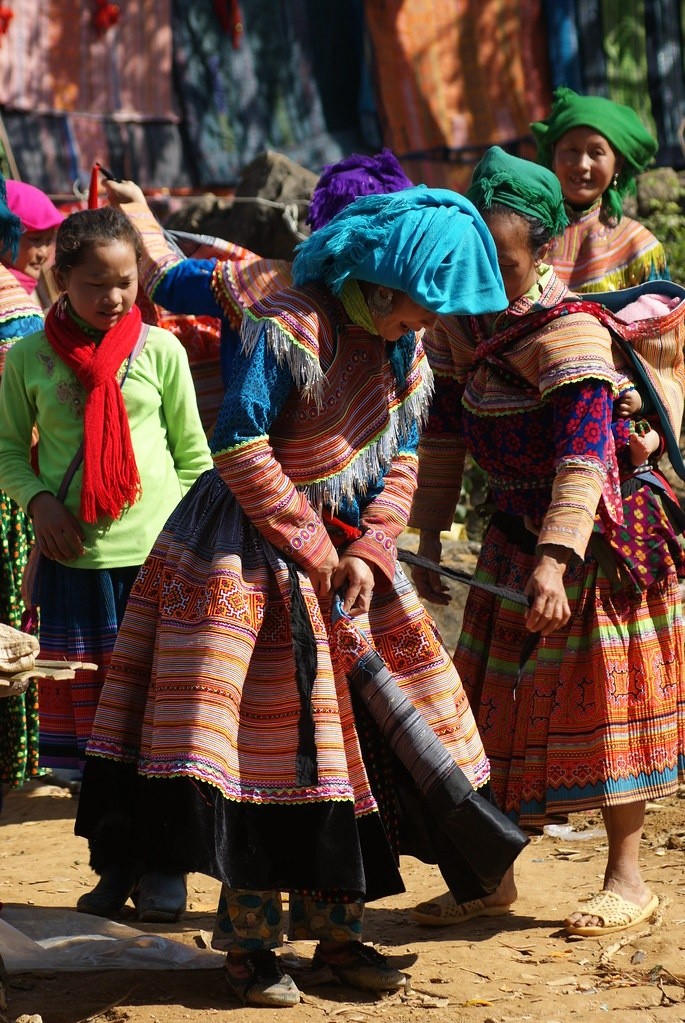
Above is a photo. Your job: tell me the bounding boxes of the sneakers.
[132,872,189,922]
[75,873,137,917]
[221,951,301,1006]
[308,946,407,992]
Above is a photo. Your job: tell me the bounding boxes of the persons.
[1,204,219,923]
[405,142,684,936]
[101,145,416,394]
[525,84,676,298]
[70,182,530,1007]
[609,293,685,469]
[1,176,70,797]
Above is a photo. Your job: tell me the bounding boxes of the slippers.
[409,890,511,927]
[564,890,659,937]
